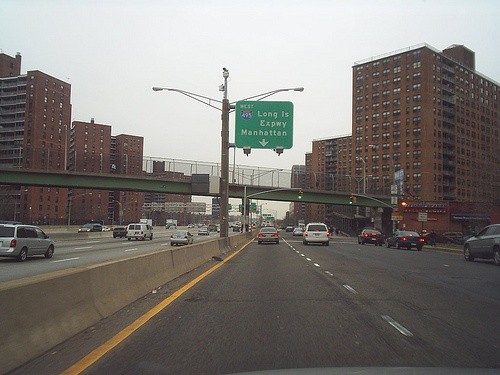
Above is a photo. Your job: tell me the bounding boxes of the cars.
[112,227,127,238]
[464,224,500,265]
[170,230,193,246]
[164,223,176,229]
[258,227,280,244]
[442,232,464,243]
[187,220,280,239]
[420,231,449,246]
[301,223,330,245]
[285,223,306,237]
[153,67,305,239]
[357,229,383,246]
[387,231,424,251]
[102,226,111,232]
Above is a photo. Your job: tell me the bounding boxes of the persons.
[335,226,339,235]
[428,229,436,246]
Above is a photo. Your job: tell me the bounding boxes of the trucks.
[139,219,152,226]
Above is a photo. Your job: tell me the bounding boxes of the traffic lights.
[298,192,301,199]
[301,191,303,198]
[349,197,354,206]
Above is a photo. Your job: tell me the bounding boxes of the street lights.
[346,174,373,230]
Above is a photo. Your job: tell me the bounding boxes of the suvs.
[0,223,55,262]
[77,224,102,232]
[126,224,154,241]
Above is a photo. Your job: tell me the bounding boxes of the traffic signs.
[236,101,294,150]
